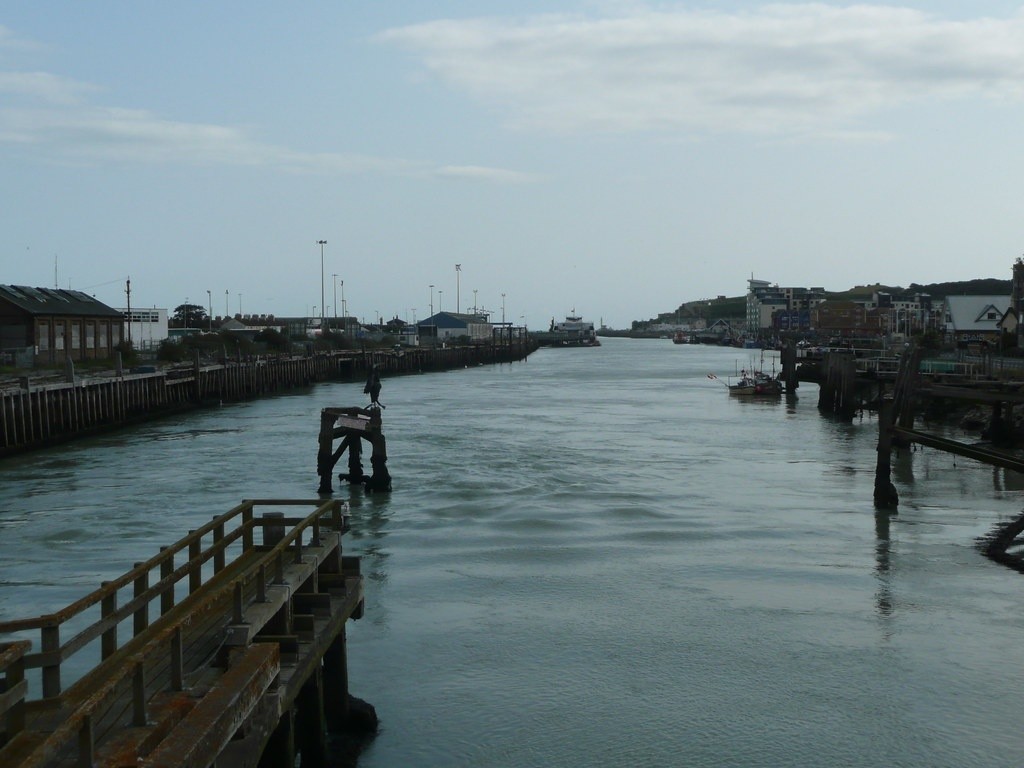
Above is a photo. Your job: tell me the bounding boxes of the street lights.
[430,264,508,327]
[316,239,327,317]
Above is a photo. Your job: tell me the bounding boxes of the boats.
[672,319,812,348]
[706,348,778,395]
